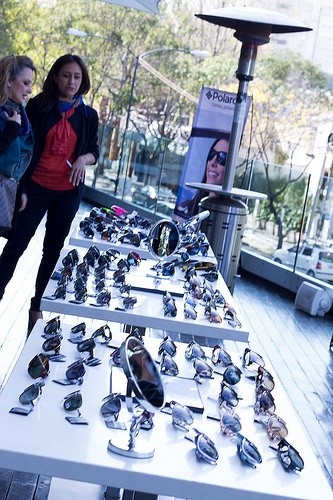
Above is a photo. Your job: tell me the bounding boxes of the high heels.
[28,309,43,333]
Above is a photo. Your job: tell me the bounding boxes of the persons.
[137,350,163,408]
[177,137,229,216]
[0,55,37,239]
[0,55,100,332]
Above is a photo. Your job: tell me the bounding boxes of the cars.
[132,183,178,214]
[274,242,333,282]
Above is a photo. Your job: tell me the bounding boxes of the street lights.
[65,27,211,196]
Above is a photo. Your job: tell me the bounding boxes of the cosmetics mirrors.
[108,337,167,458]
[146,219,179,280]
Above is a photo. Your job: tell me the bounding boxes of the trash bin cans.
[198,196,249,295]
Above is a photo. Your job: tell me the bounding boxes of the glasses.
[64,390,82,416]
[160,400,194,432]
[42,244,242,328]
[65,359,86,386]
[207,149,227,166]
[254,409,288,441]
[184,427,219,465]
[76,337,96,359]
[90,324,112,344]
[134,404,155,430]
[70,322,86,338]
[79,205,210,257]
[27,353,50,379]
[19,380,45,407]
[230,432,262,469]
[101,392,122,421]
[42,334,63,356]
[269,438,304,473]
[109,328,276,416]
[206,400,241,436]
[44,316,62,335]
